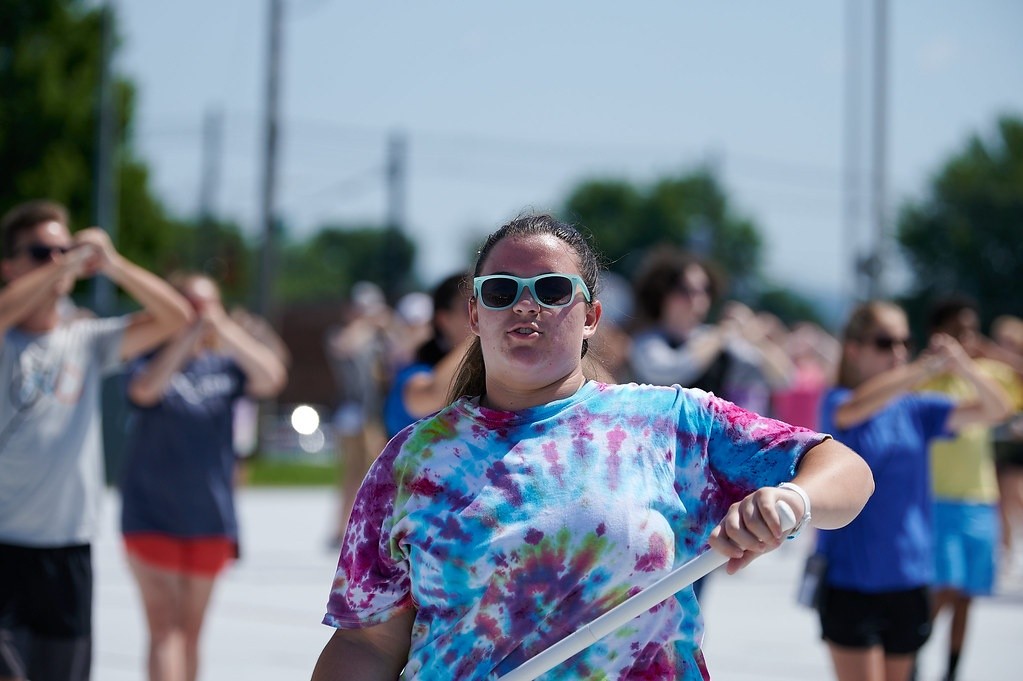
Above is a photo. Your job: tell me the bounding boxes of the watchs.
[777,482,812,540]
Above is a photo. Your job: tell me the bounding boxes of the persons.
[311,215,876,681]
[329,269,478,545]
[586,258,1023,681]
[0,206,286,681]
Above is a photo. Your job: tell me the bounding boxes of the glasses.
[10,244,70,265]
[473,272,591,310]
[863,337,909,354]
